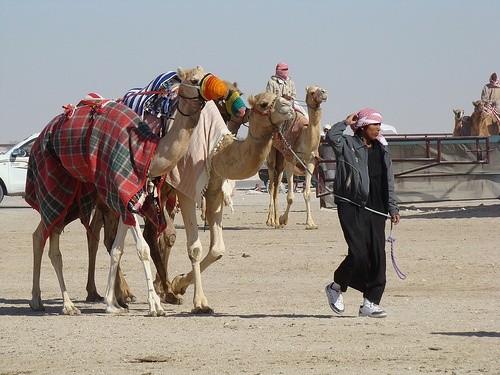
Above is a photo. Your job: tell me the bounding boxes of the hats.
[350,109,382,132]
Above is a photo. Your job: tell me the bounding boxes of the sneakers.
[325,281,345,314]
[358,302,387,318]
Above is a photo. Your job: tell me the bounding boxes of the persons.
[264,60,299,110]
[481,71,500,114]
[324,108,401,318]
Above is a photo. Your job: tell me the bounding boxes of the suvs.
[257,158,320,187]
[0,133,40,207]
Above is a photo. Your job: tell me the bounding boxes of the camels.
[265,84,328,230]
[453,100,500,136]
[29,65,294,316]
